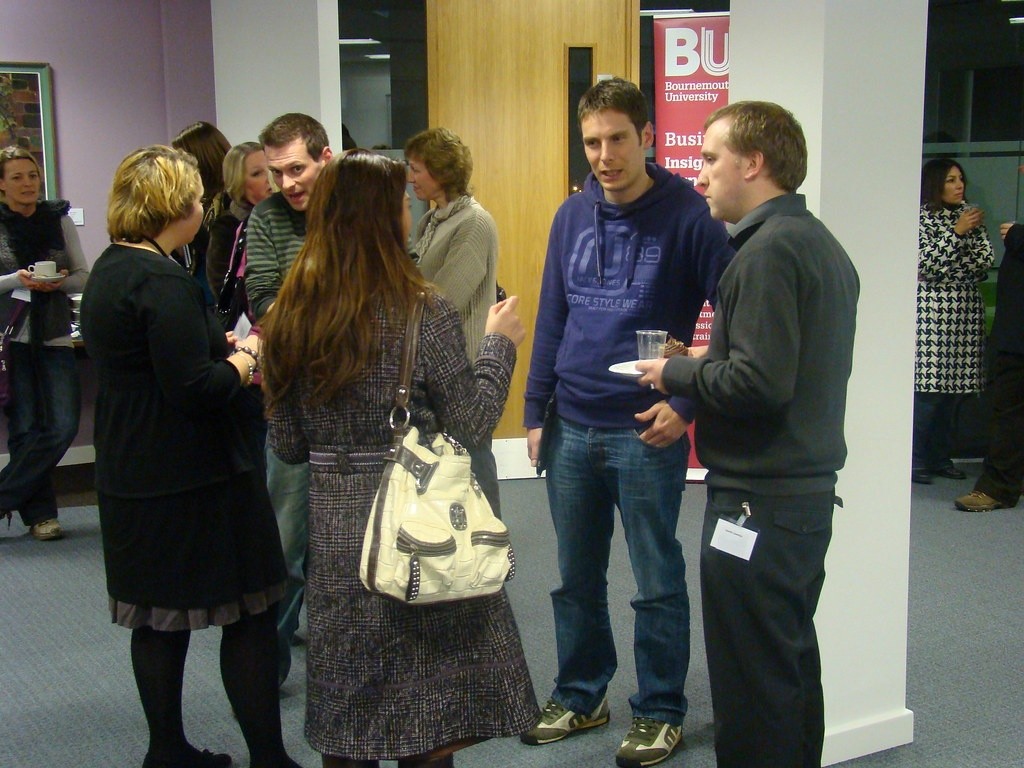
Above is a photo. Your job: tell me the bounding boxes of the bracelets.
[238,353,253,384]
[230,347,260,371]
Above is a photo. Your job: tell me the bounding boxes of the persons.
[172,113,499,685]
[633,101,861,768]
[521,77,732,768]
[911,157,994,483]
[78,145,297,767]
[262,148,543,768]
[954,163,1024,510]
[0,144,87,537]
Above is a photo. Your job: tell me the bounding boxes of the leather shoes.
[931,463,965,479]
[913,465,931,484]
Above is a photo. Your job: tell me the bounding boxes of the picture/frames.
[0,61,58,206]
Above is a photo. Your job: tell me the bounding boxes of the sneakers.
[614,703,681,768]
[955,489,1011,512]
[518,700,610,745]
[31,518,62,540]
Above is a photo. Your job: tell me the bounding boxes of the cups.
[635,329,668,361]
[28,261,56,277]
[963,203,979,214]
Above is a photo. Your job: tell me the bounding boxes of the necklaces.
[143,237,169,257]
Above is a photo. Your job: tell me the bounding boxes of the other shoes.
[144,742,231,768]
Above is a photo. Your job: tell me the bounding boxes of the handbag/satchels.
[359,297,512,605]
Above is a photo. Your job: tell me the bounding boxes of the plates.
[608,358,666,378]
[32,273,66,282]
